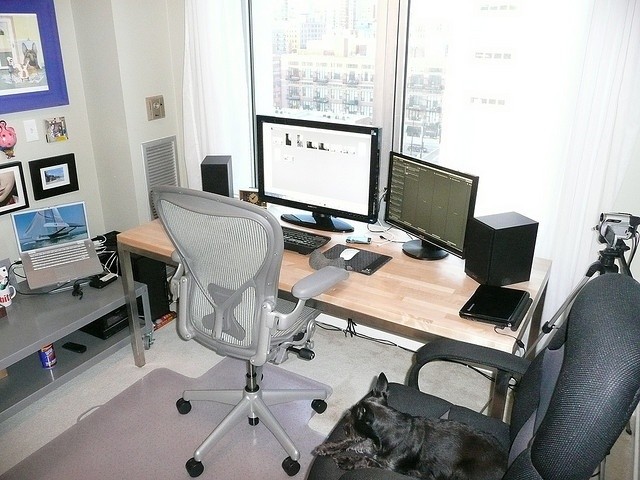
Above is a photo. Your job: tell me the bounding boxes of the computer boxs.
[90,229,171,321]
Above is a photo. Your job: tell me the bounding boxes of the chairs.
[306,272,638,479]
[151,184,351,478]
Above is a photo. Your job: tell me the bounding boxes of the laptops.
[9,201,104,292]
[459,285,533,330]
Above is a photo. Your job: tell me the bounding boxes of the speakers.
[200,155,234,198]
[464,211,540,286]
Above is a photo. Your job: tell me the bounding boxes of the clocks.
[239,187,259,206]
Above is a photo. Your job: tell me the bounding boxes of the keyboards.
[280,225,331,255]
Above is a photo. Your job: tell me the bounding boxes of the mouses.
[339,248,360,261]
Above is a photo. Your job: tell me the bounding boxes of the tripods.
[479,249,632,480]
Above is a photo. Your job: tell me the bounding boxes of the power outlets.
[0,259,17,286]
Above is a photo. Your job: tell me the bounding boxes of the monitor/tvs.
[257,114,383,233]
[384,151,480,261]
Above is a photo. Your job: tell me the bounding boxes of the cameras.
[590,209,640,251]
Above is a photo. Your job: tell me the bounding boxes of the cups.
[0,283,17,308]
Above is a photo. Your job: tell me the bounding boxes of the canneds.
[39,344,58,368]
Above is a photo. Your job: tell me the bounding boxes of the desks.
[0,273,156,425]
[115,194,553,422]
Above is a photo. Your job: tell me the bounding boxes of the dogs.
[311,372,509,480]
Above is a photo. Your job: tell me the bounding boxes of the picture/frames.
[0,0,70,116]
[28,153,79,200]
[0,161,29,216]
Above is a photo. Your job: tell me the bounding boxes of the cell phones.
[62,341,87,353]
